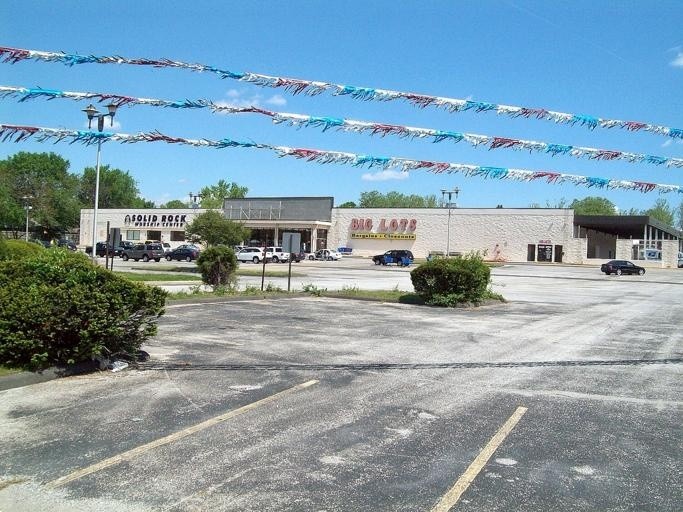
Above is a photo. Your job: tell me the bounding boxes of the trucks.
[645,249,683,268]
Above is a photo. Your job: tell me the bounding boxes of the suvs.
[371,249,415,267]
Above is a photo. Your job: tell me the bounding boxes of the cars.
[306,248,343,261]
[600,259,646,276]
[36,238,307,265]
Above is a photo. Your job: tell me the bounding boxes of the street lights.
[82,99,119,259]
[440,186,462,258]
[22,195,34,244]
[188,190,203,214]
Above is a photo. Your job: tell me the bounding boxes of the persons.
[319,239,324,249]
[49,235,60,247]
